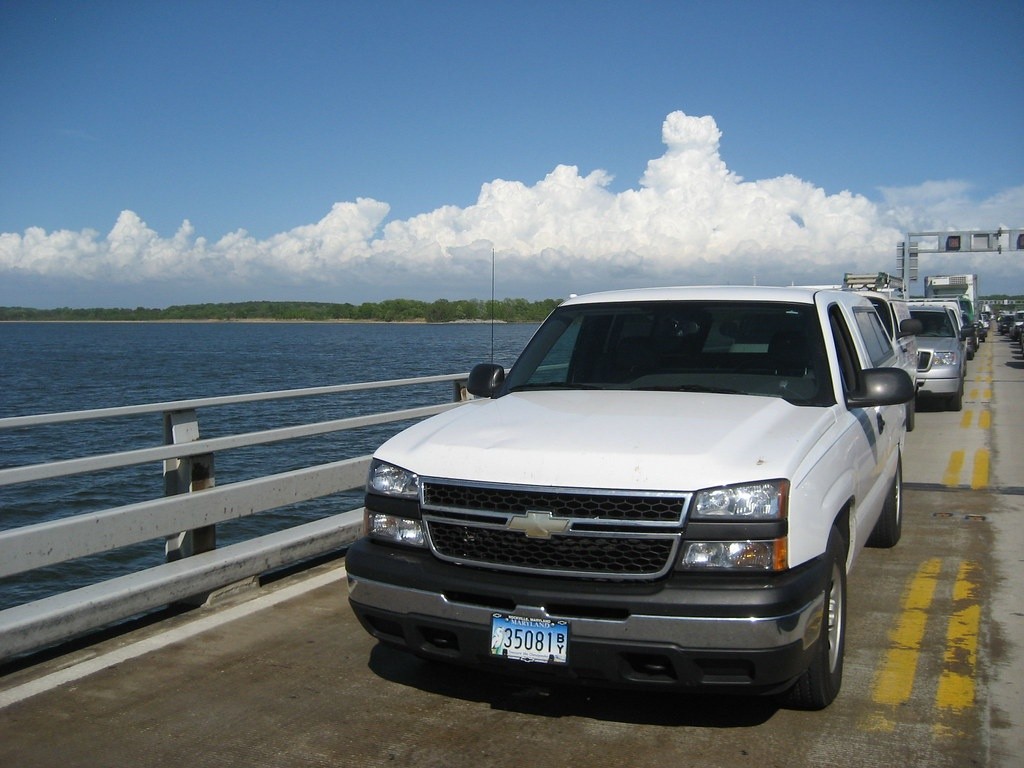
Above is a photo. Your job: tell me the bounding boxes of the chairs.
[611,336,663,383]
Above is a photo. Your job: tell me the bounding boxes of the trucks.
[924,273,978,360]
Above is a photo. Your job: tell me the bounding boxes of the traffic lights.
[1017,233,1024,249]
[947,236,961,250]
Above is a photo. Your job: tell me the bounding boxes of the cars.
[978,304,992,343]
[995,309,1024,354]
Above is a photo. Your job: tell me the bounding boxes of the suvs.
[344,283,916,711]
[787,272,976,411]
